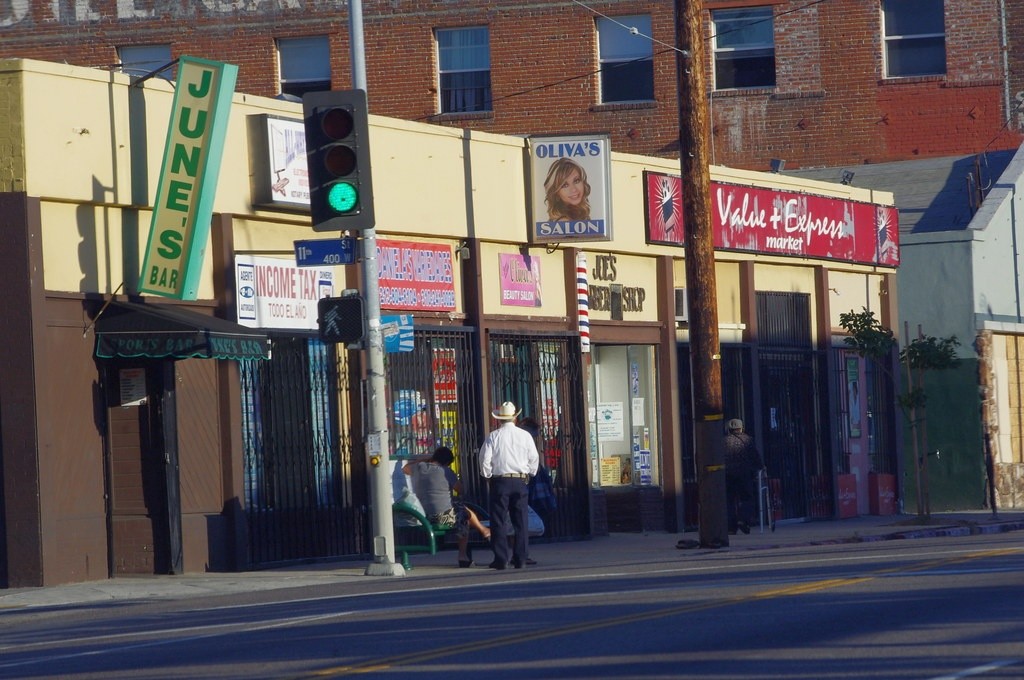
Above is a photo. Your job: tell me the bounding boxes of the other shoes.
[489,562,505,569]
[511,560,524,568]
[526,558,537,564]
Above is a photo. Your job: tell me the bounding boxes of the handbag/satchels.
[393,486,425,527]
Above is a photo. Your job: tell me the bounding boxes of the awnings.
[93,300,272,361]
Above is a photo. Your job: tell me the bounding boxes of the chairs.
[387,503,451,571]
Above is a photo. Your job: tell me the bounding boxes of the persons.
[403,446,491,568]
[623,458,631,484]
[723,419,762,535]
[478,402,539,570]
[850,382,860,424]
[542,157,592,222]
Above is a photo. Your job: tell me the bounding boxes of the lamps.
[840,170,855,185]
[770,158,785,174]
[455,242,470,262]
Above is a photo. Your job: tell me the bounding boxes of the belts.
[493,473,527,479]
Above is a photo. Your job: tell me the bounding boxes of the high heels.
[485,536,491,543]
[459,559,470,567]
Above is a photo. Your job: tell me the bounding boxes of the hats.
[728,419,743,429]
[492,402,522,420]
